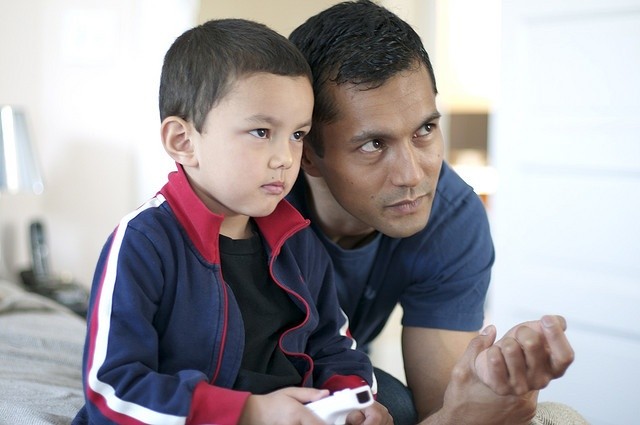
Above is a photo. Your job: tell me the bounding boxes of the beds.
[1,270,94,425]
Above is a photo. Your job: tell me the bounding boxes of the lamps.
[1,101,46,266]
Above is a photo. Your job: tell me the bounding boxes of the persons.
[83,16,395,424]
[67,0,577,425]
